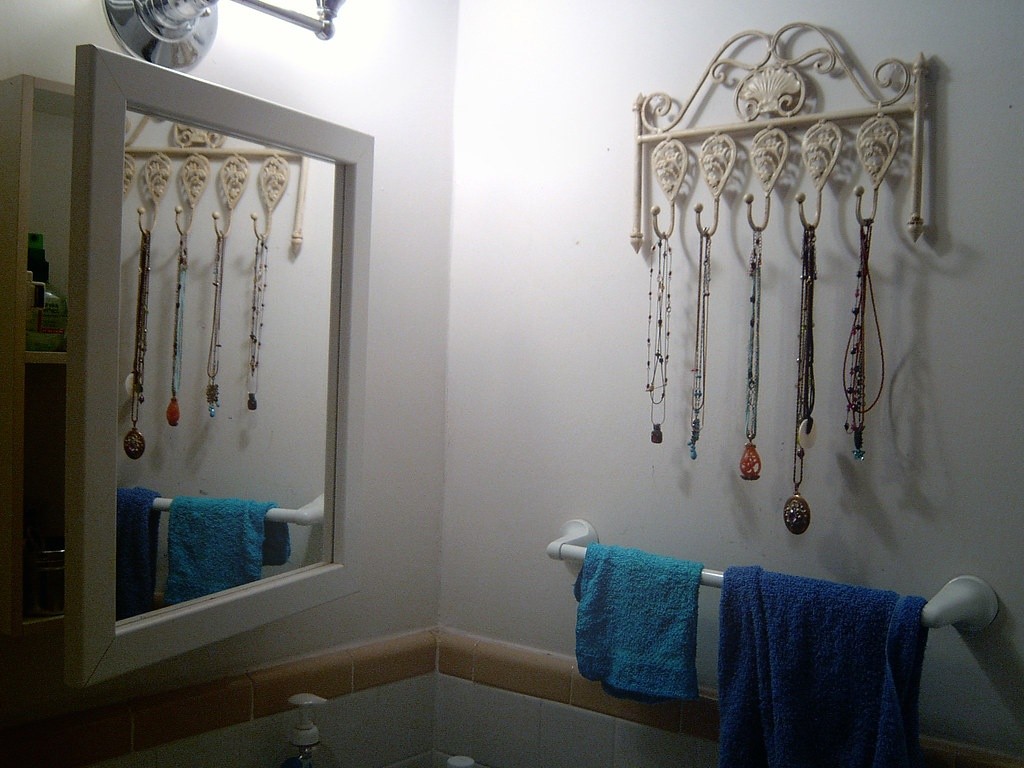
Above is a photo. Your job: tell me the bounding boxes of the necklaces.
[646,231,672,444]
[123,229,152,460]
[842,217,885,461]
[247,233,269,411]
[206,230,226,417]
[783,224,817,535]
[687,227,712,460]
[739,226,763,481]
[166,231,188,427]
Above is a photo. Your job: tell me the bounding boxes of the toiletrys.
[28,233,67,351]
[276,694,339,768]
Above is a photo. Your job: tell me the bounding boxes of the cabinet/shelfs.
[0,75,74,638]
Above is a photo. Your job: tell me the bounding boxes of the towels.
[572,540,706,706]
[718,564,928,768]
[164,496,291,605]
[116,487,162,624]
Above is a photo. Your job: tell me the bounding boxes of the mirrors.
[63,44,373,688]
